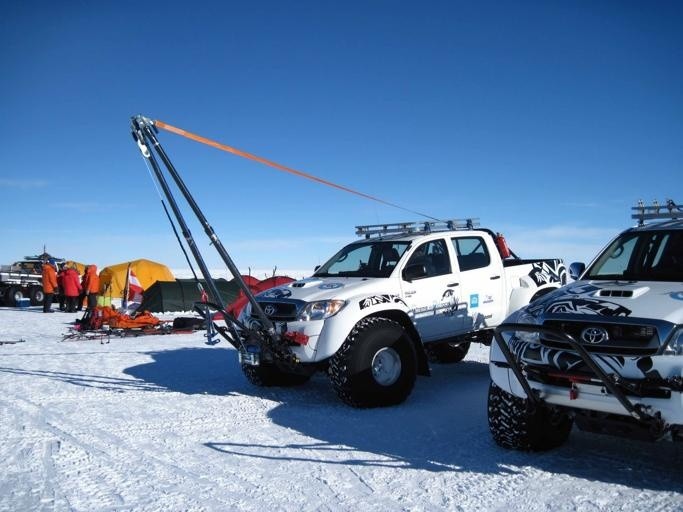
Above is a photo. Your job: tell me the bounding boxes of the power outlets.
[231,219,570,407]
[1,257,82,309]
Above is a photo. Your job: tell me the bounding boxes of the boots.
[485,200,682,463]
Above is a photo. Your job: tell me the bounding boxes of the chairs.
[42,306,82,314]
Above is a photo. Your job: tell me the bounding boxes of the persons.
[41,259,58,312]
[57,261,100,313]
[31,268,37,274]
